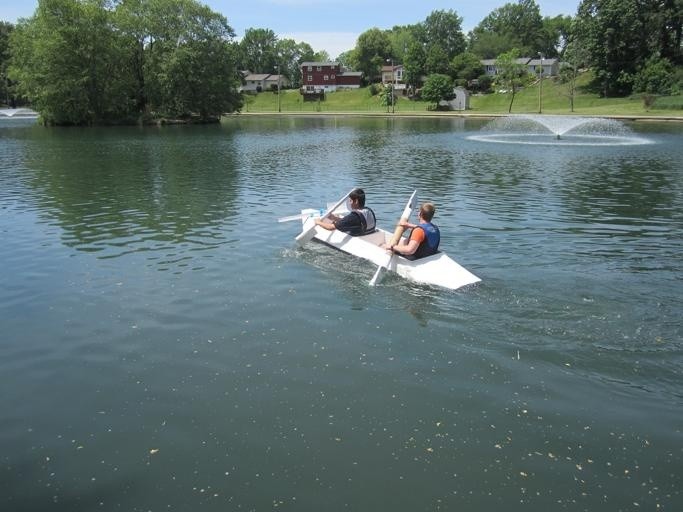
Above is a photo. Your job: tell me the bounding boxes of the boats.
[300,202,485,296]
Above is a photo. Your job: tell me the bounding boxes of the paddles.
[294,187,356,248]
[369,189,418,287]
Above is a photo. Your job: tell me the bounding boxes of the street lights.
[257,50,281,113]
[537,52,543,114]
[386,58,395,113]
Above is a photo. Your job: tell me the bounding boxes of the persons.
[384,203,440,261]
[314,188,376,236]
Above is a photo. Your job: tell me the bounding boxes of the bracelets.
[391,245,394,251]
